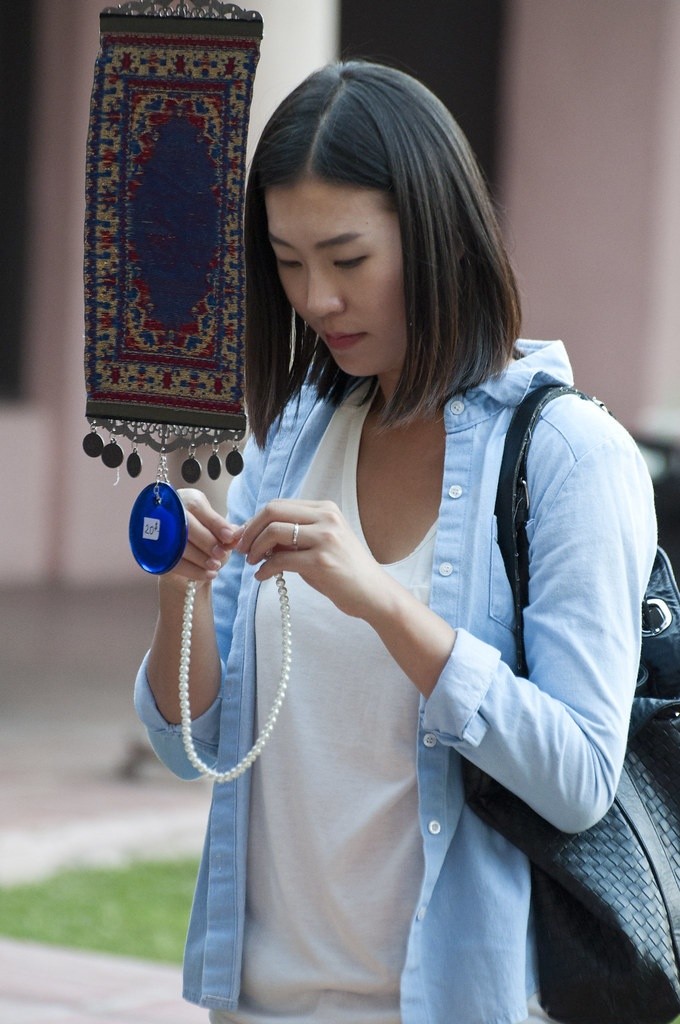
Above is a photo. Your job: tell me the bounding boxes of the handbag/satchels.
[465,383,680,1023]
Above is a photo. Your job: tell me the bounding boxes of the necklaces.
[178,534,293,782]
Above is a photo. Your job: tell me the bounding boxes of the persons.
[124,54,664,1024]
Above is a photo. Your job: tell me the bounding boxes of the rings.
[292,522,300,553]
